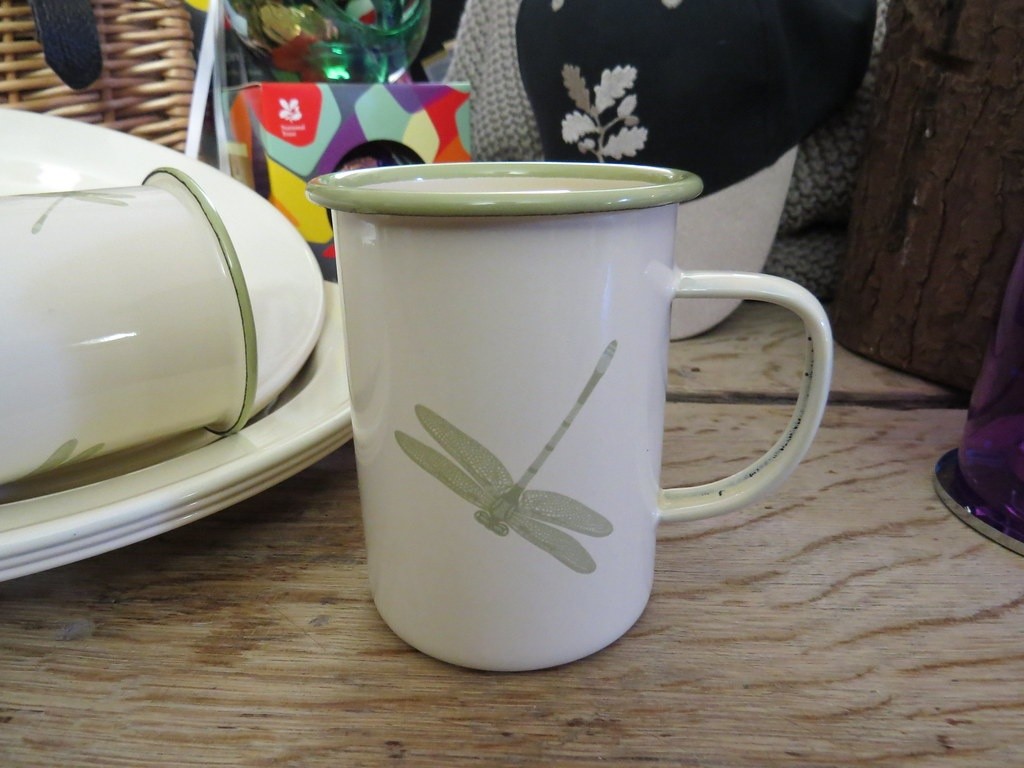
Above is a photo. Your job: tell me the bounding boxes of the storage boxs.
[221,81,470,283]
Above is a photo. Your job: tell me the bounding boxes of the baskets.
[0,0,195,153]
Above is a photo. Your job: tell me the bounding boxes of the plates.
[0,107,325,418]
[0,279,353,582]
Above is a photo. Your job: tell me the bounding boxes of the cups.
[305,163,835,672]
[0,167,257,486]
[933,241,1024,556]
